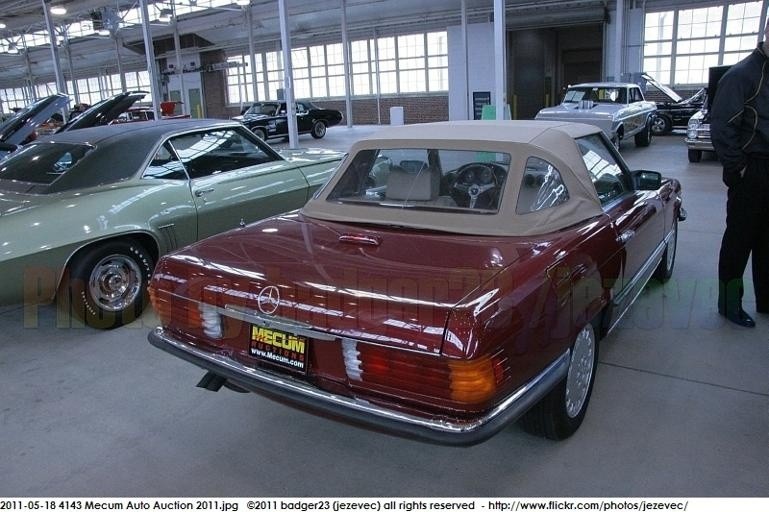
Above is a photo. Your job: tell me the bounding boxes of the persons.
[710,17,769,329]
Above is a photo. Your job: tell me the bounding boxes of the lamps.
[50,4,66,14]
[159,9,173,21]
[8,43,19,54]
[0,19,7,28]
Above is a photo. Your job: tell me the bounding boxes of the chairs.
[384,166,459,207]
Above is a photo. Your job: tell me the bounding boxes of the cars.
[146,120,683,451]
[538,74,718,163]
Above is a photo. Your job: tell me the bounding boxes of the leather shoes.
[719,299,769,327]
